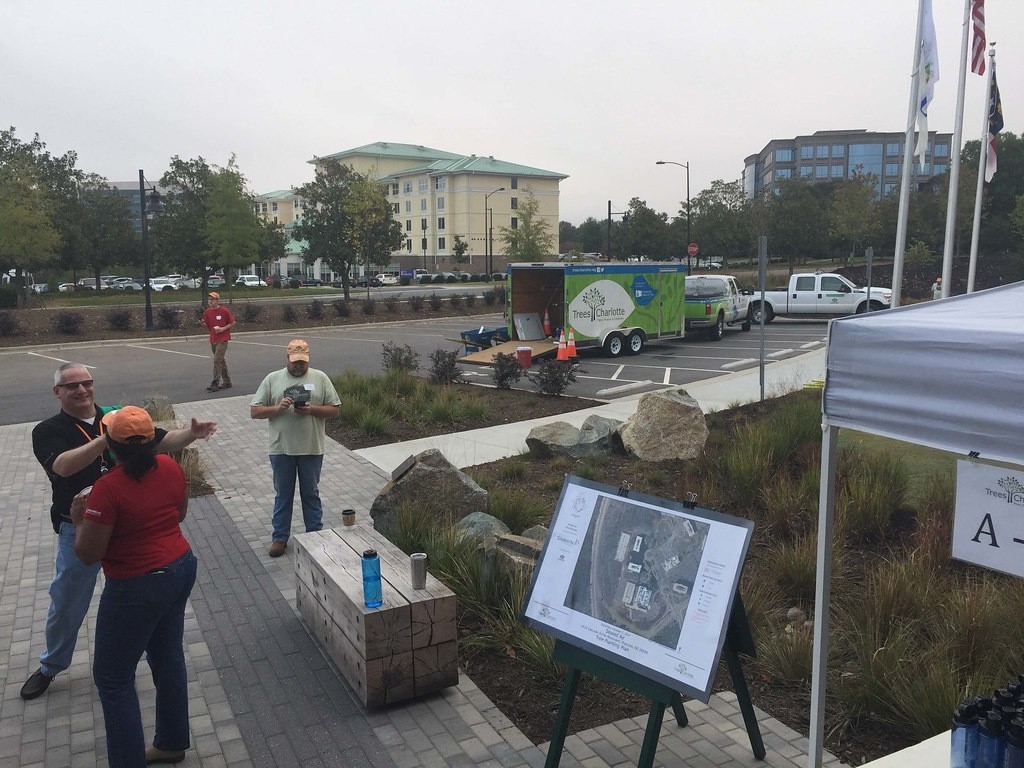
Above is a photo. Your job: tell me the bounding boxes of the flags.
[913,0,940,174]
[970,0,987,76]
[985,59,1004,183]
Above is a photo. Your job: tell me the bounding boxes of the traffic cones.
[555,330,570,360]
[568,327,577,358]
[543,310,552,335]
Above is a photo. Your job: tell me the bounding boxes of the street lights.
[487,208,492,276]
[485,187,505,274]
[424,226,430,269]
[656,160,691,275]
[607,211,629,261]
[140,185,167,330]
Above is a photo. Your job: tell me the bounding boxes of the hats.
[286,340,310,362]
[209,292,220,299]
[103,406,155,444]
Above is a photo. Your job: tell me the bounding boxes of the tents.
[807,281,1024,768]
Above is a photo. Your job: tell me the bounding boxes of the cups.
[342,509,356,530]
[410,553,427,589]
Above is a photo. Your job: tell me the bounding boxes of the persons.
[931,278,942,301]
[71,406,198,768]
[250,339,342,557]
[198,291,236,391]
[20,364,218,699]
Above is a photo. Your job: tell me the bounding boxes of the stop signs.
[687,243,699,256]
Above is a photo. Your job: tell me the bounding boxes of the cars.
[3,266,327,296]
[330,269,428,288]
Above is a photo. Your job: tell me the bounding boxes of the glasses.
[56,379,94,391]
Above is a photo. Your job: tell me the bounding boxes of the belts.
[60,515,73,523]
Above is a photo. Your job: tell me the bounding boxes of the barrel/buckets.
[516,346,533,368]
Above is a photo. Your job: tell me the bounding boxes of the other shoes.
[20,665,56,700]
[269,540,288,557]
[219,382,232,389]
[145,745,185,765]
[206,382,218,391]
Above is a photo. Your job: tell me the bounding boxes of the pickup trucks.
[683,273,755,340]
[743,271,892,325]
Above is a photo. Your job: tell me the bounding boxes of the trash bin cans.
[516,346,533,369]
[99,406,124,462]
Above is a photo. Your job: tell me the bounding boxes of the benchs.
[293,521,461,712]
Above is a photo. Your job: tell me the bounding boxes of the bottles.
[361,550,382,607]
[950,671,1024,768]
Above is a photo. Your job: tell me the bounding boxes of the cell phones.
[294,401,305,410]
